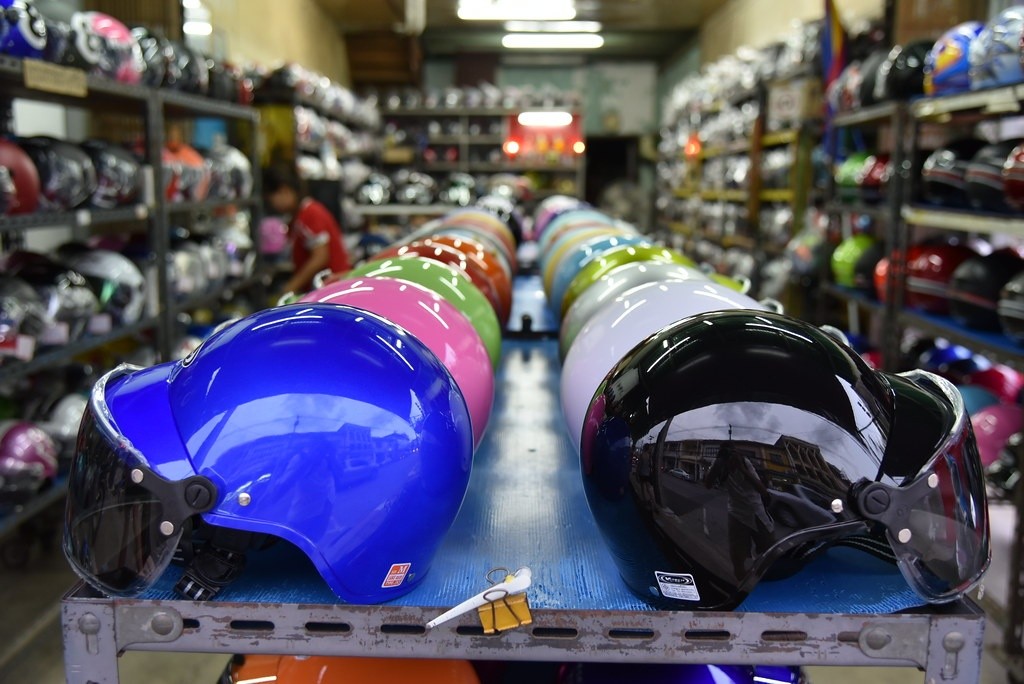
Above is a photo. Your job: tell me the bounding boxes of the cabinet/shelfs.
[255,97,368,232]
[0,55,264,670]
[818,103,896,368]
[378,109,585,211]
[899,85,1024,661]
[653,67,822,289]
[60,268,985,684]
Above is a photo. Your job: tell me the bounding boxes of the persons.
[258,161,347,297]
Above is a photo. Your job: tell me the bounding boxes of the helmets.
[532,192,990,684]
[647,0,1024,481]
[0,0,373,509]
[62,194,517,684]
